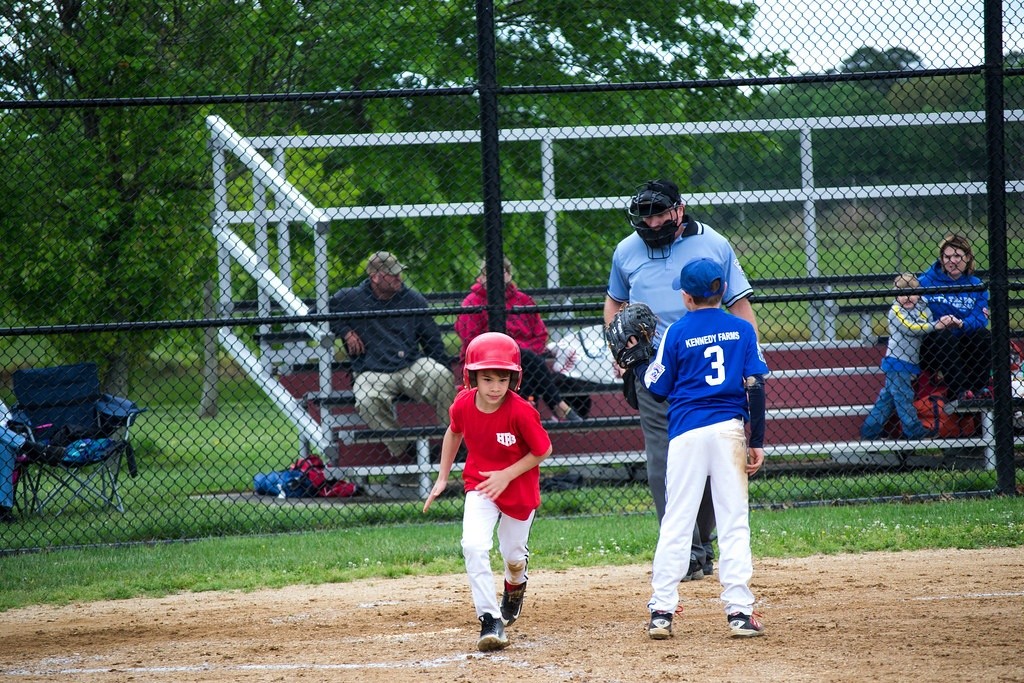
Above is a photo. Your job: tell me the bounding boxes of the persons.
[1010,339,1024,399]
[917,235,993,399]
[603,180,759,581]
[0,397,18,522]
[329,251,456,485]
[859,273,948,440]
[423,332,552,652]
[606,258,769,639]
[457,254,583,422]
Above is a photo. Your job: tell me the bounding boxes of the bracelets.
[958,318,963,328]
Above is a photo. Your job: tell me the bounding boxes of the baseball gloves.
[603,301,661,371]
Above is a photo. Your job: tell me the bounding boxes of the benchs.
[229,287,643,501]
[828,276,1024,469]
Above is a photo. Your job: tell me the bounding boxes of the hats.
[672,257,726,295]
[366,251,409,276]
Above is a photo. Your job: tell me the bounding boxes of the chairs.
[13,363,144,512]
[0,396,54,528]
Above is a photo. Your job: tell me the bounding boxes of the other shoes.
[957,390,975,408]
[916,431,940,440]
[399,457,420,488]
[550,408,582,423]
[702,560,713,575]
[875,431,890,440]
[977,387,993,407]
[680,561,704,581]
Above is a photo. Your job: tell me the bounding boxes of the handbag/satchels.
[254,454,361,497]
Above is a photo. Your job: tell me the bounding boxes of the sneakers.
[477,613,508,651]
[498,578,528,627]
[648,604,683,639]
[727,611,765,638]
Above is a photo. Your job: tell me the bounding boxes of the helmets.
[463,332,523,393]
[629,180,682,258]
